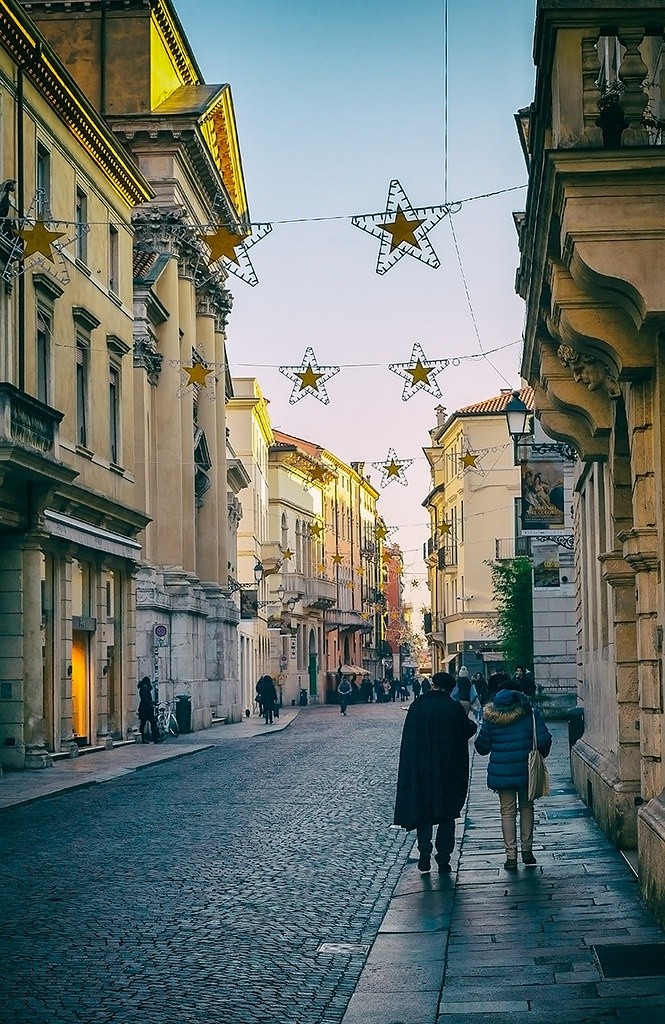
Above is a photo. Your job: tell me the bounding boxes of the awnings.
[441,654,458,663]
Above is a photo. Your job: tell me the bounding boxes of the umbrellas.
[349,665,371,677]
[327,665,359,680]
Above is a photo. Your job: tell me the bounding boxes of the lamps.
[256,585,285,609]
[228,560,265,593]
[281,597,296,615]
[456,593,475,602]
[500,391,578,466]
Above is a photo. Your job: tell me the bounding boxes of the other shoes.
[417,859,431,871]
[142,739,149,743]
[437,863,452,873]
[504,859,517,868]
[154,738,165,743]
[523,851,536,865]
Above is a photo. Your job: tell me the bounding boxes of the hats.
[431,673,456,690]
[459,666,468,677]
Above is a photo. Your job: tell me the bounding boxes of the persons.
[256,675,277,724]
[351,676,356,688]
[428,677,433,685]
[338,677,352,716]
[373,677,396,703]
[421,678,431,693]
[137,677,166,744]
[475,679,552,870]
[451,666,509,725]
[515,666,530,696]
[393,673,477,872]
[360,679,374,703]
[413,680,421,699]
[390,678,410,702]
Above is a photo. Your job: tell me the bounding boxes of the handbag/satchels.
[274,702,279,718]
[528,750,550,801]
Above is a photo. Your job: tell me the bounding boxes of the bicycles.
[143,697,180,744]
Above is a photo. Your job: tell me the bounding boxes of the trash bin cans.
[174,695,193,735]
[300,688,308,706]
